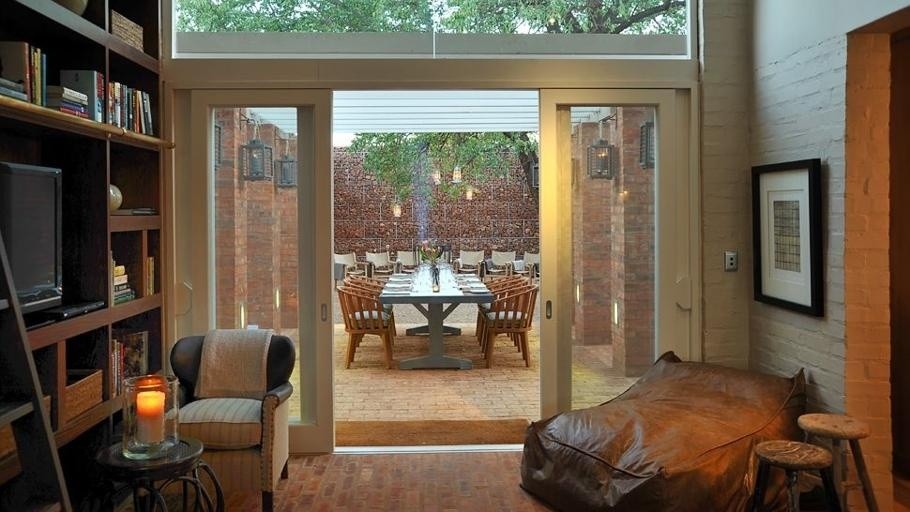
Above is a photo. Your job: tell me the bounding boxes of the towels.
[190,326,277,399]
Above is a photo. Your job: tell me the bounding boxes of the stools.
[797,410,880,510]
[747,440,839,510]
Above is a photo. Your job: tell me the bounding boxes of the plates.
[457,269,489,294]
[382,269,416,294]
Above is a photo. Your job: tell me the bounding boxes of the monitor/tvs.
[0,161,63,330]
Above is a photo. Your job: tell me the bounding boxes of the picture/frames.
[747,156,825,322]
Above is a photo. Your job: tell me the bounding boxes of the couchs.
[517,349,808,512]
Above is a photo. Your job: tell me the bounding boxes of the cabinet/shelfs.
[0,0,179,512]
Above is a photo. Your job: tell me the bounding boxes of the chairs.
[330,243,540,372]
[160,326,297,512]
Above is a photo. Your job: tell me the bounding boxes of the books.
[0,42,154,136]
[111,251,154,401]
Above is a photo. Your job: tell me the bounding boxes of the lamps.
[234,113,276,185]
[637,122,657,169]
[274,134,301,188]
[587,120,618,179]
[214,119,223,172]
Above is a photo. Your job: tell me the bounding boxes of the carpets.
[328,415,533,447]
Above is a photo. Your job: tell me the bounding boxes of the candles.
[136,392,170,447]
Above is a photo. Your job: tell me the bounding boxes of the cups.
[121,374,180,460]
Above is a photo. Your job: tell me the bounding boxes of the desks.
[89,434,227,510]
